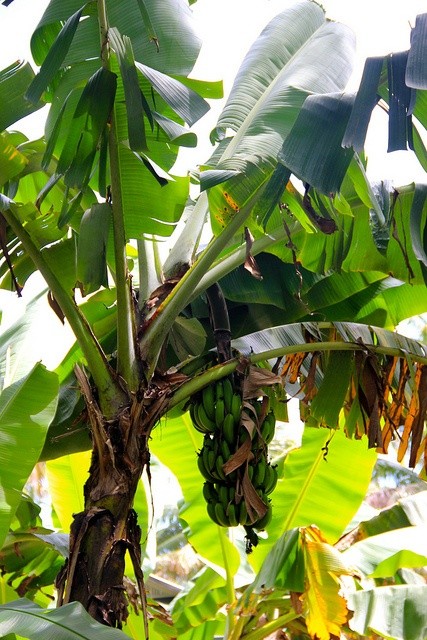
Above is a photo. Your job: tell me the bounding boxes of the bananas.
[188,378,278,530]
